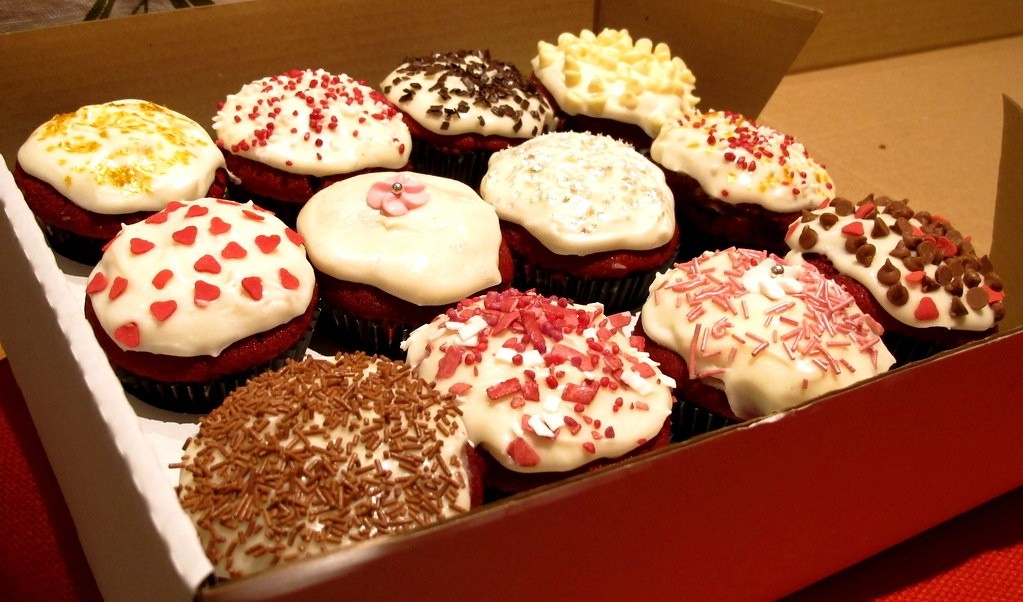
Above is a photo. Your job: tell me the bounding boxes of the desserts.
[15,25,1006,588]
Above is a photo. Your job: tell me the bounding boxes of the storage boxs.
[0,0,1023,602]
